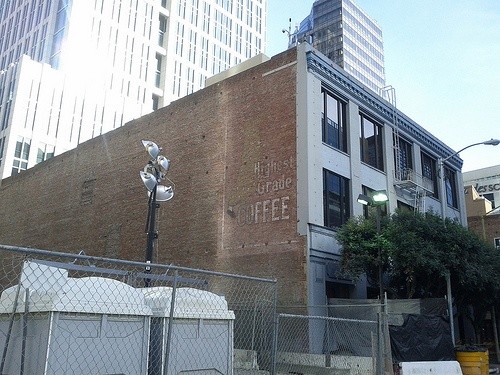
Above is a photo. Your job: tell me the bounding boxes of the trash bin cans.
[456,345,489,375]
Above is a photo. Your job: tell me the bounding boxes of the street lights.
[438,137,500,347]
[357,189,389,305]
